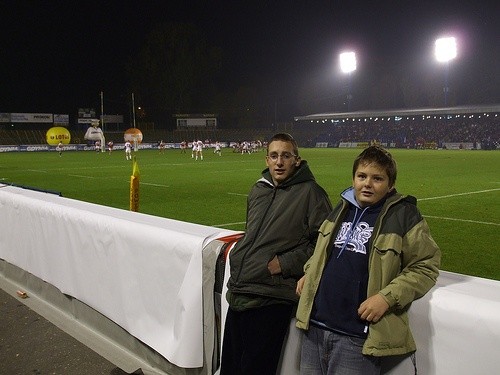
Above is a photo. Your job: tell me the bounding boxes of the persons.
[54,138,264,162]
[219,133,334,375]
[294,144,441,375]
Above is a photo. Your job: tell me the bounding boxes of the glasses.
[266,153,297,161]
